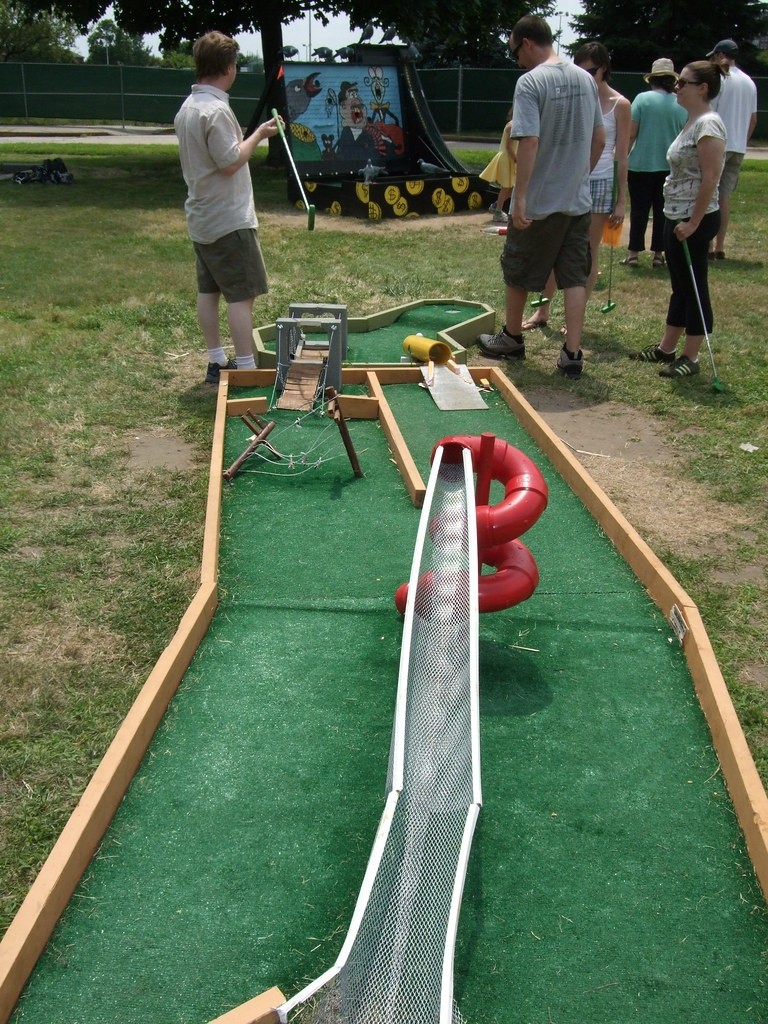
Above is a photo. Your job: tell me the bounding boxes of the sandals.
[653,256,664,269]
[619,256,638,268]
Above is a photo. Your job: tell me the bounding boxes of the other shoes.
[708,252,715,260]
[715,251,724,260]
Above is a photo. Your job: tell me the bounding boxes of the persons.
[613,58,690,267]
[174,30,286,383]
[478,108,520,223]
[475,16,606,380]
[521,42,632,331]
[706,41,758,259]
[627,60,730,379]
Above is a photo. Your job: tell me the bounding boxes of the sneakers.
[659,355,701,379]
[629,342,677,364]
[205,358,237,383]
[488,204,496,214]
[493,212,508,222]
[477,325,525,361]
[557,342,583,380]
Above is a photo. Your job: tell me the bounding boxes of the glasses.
[586,64,603,76]
[509,43,523,63]
[673,78,698,89]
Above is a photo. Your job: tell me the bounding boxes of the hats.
[643,58,680,86]
[706,39,739,56]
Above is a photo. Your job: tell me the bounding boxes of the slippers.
[520,318,547,330]
[559,325,568,335]
